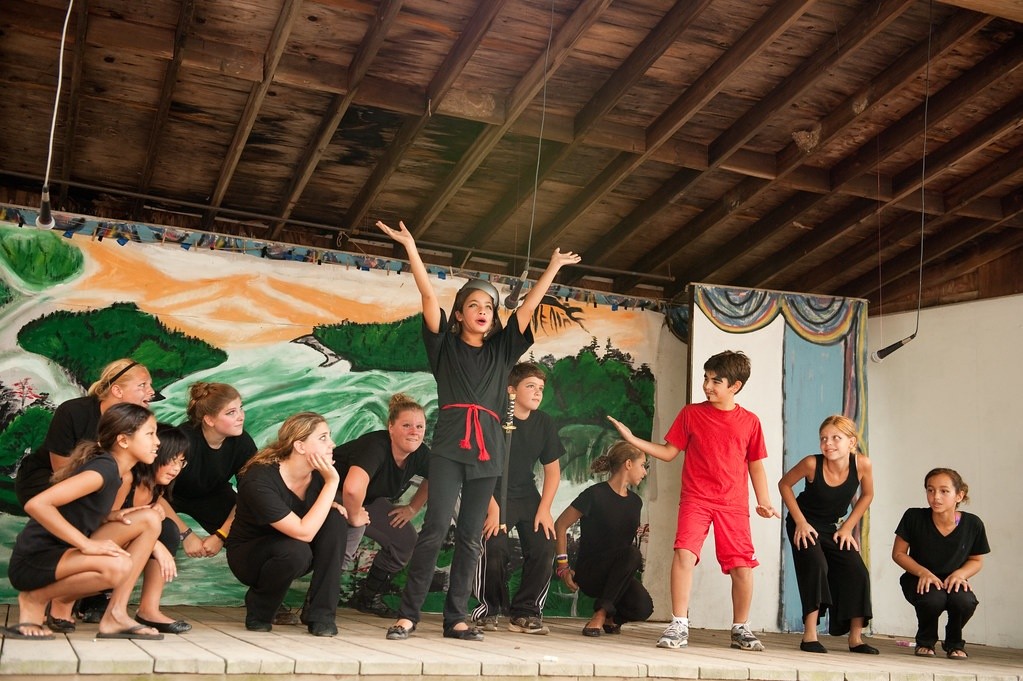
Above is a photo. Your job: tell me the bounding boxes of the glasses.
[168,457,187,469]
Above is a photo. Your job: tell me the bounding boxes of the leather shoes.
[47,607,76,633]
[134,610,193,632]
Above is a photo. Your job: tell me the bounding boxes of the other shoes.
[245,606,272,631]
[385,619,413,640]
[442,626,485,640]
[582,621,600,636]
[848,643,879,654]
[82,607,104,623]
[801,639,827,653]
[601,623,621,634]
[299,613,339,637]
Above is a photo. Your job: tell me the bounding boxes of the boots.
[356,564,398,618]
[301,568,345,623]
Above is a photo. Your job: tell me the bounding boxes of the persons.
[0,359,192,640]
[299,391,433,625]
[778,415,880,655]
[554,440,654,636]
[467,362,567,633]
[377,219,581,640]
[607,351,781,651]
[79,382,301,622]
[224,410,348,637]
[892,466,991,660]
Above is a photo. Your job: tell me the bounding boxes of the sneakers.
[271,603,299,625]
[730,620,764,651]
[474,614,499,631]
[655,613,689,648]
[507,615,549,635]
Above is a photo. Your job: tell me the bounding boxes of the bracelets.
[215,529,227,541]
[556,554,575,578]
[408,505,415,513]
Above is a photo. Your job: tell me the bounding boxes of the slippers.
[0,622,55,640]
[95,624,164,640]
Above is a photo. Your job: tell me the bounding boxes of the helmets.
[459,279,499,307]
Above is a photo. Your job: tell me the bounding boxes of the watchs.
[180,528,192,541]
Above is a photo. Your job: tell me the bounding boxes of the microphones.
[871,334,916,364]
[505,271,529,309]
[35,186,55,230]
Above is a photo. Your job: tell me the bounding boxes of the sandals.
[946,649,969,659]
[914,645,936,657]
